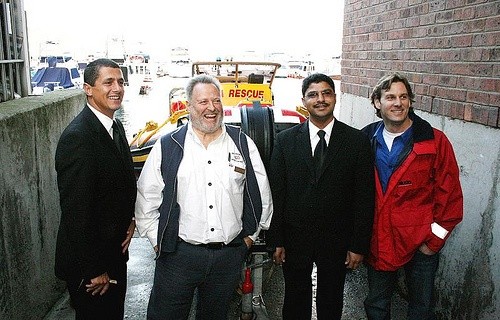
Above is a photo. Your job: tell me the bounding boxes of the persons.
[53,58,136,320]
[133,75,273,320]
[361,72,463,320]
[269,73,376,320]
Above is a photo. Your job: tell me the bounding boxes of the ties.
[112,121,125,159]
[313,130,327,173]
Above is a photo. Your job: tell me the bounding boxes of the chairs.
[248,74,264,84]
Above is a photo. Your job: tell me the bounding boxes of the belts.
[198,242,243,250]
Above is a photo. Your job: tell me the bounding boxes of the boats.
[127,55,311,173]
[29,37,342,106]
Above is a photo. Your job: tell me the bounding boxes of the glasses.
[305,91,334,97]
